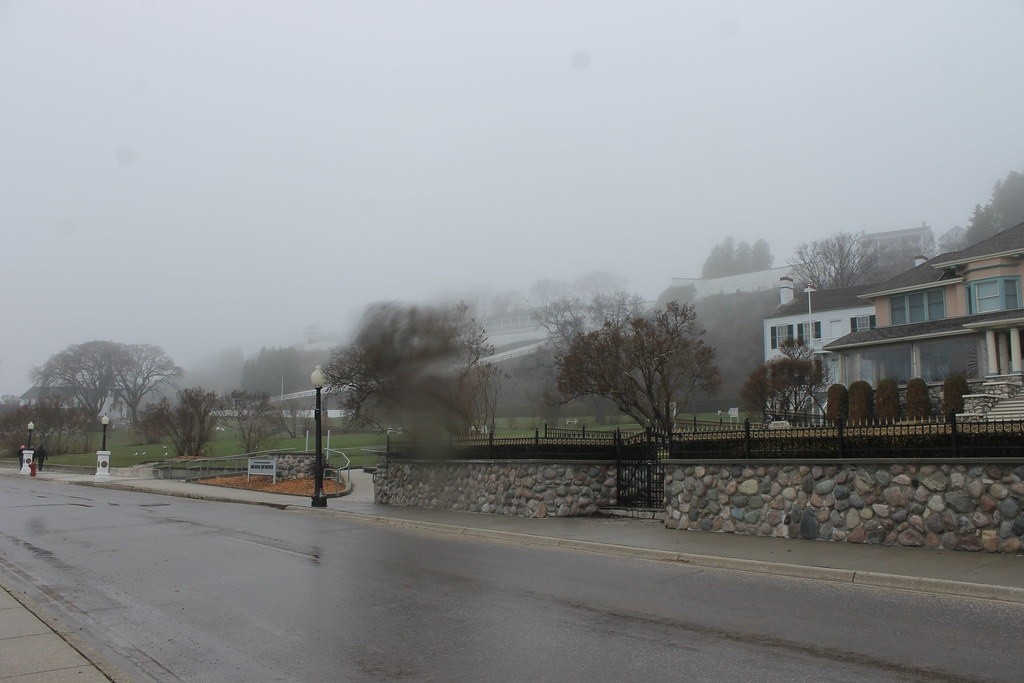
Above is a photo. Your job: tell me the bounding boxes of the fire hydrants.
[28,462,37,476]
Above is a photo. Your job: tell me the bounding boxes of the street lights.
[95,412,111,477]
[311,365,328,507]
[19,421,35,475]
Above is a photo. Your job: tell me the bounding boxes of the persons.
[17,445,26,470]
[30,445,48,471]
